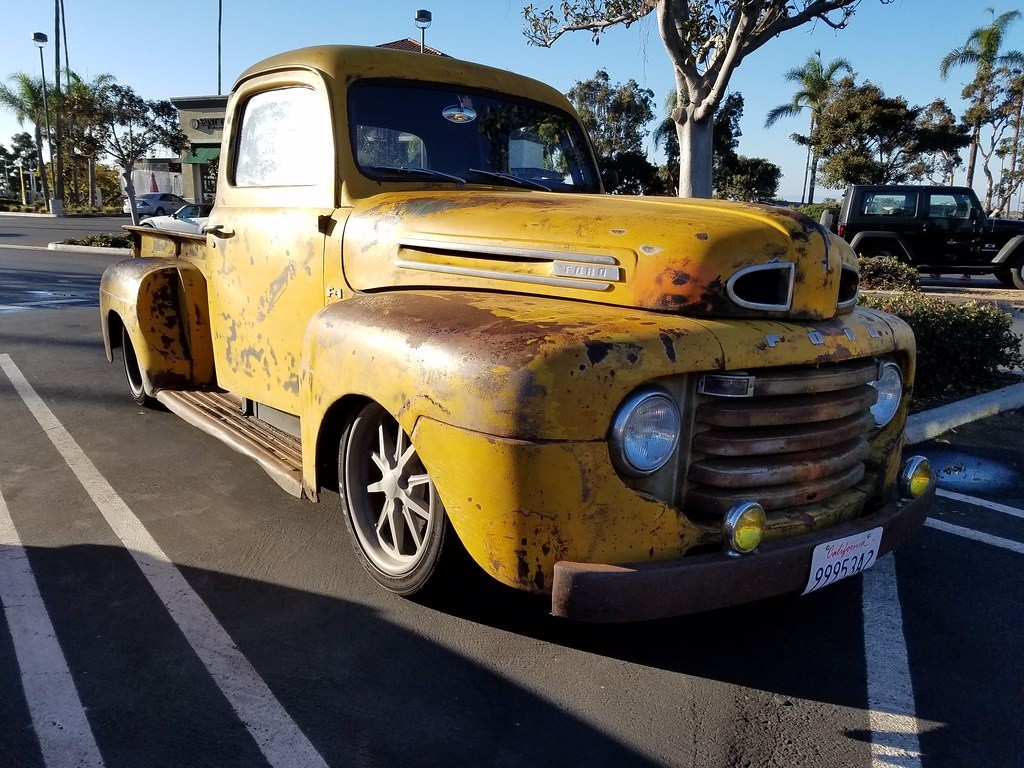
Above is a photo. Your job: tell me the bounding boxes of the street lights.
[413,8,433,52]
[96,43,936,625]
[29,29,61,199]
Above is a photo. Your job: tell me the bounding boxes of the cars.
[123,191,196,216]
[137,203,213,235]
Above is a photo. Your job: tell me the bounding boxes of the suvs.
[835,184,1024,290]
